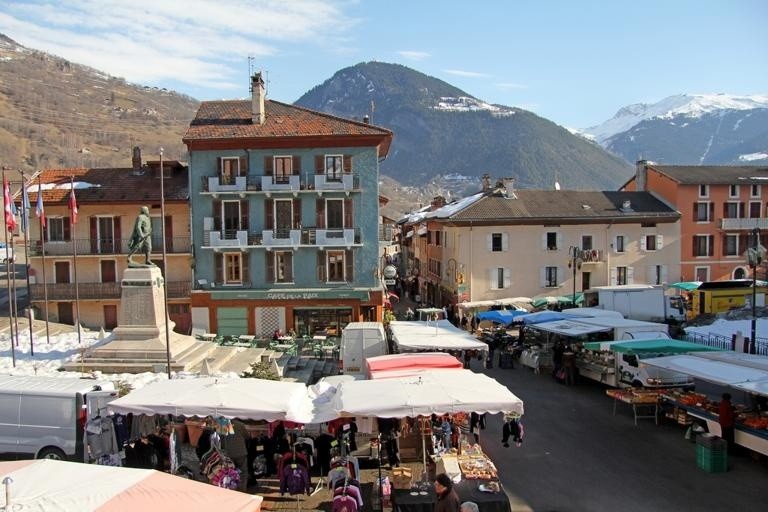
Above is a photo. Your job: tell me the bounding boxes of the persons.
[429,474,461,512]
[719,392,736,454]
[461,501,479,512]
[379,415,404,466]
[127,206,156,266]
[452,311,584,387]
[272,327,296,344]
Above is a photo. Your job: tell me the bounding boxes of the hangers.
[124,424,176,453]
[200,446,236,480]
[332,453,361,506]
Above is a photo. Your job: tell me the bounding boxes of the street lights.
[744,228,767,354]
[446,258,457,283]
[568,245,582,308]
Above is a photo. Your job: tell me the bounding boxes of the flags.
[3,175,13,231]
[68,186,78,223]
[19,182,31,233]
[8,192,18,231]
[35,180,45,227]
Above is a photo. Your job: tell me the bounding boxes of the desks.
[606,386,660,427]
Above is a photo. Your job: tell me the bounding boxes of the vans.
[339,321,389,380]
[0,373,115,461]
